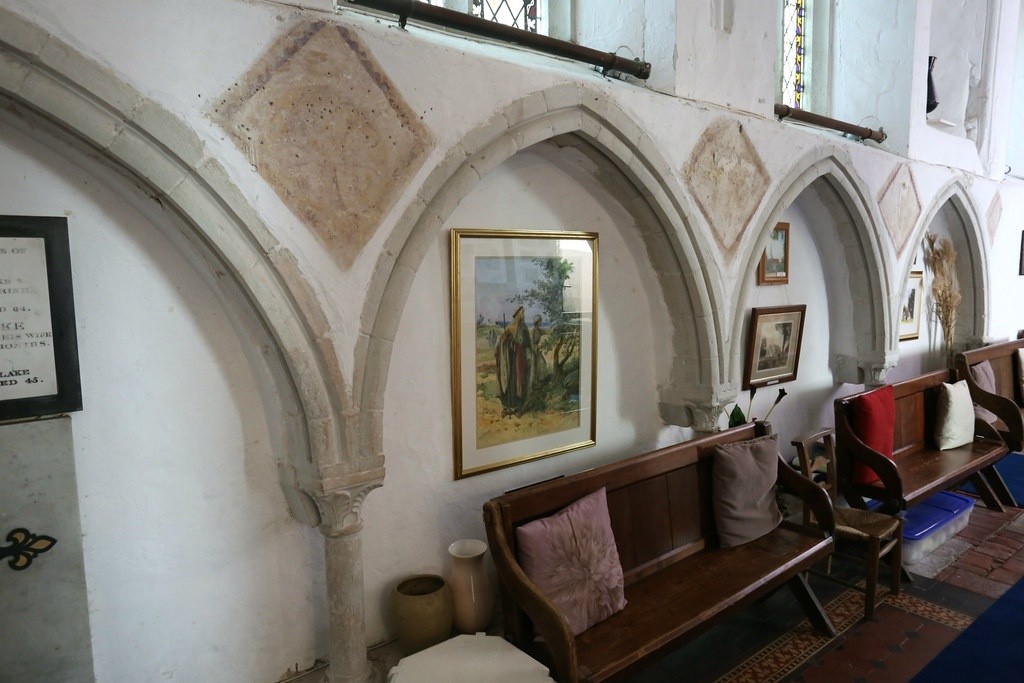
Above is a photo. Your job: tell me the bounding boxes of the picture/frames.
[898,271,924,342]
[450,227,599,480]
[743,303,807,391]
[758,222,790,284]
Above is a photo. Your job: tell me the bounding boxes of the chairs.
[791,426,903,621]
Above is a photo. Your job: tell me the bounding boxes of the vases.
[450,538,493,633]
[391,573,452,656]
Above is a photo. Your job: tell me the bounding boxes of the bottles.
[443,540,500,636]
[394,574,454,657]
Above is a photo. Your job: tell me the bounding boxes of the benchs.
[484,420,839,683]
[957,337,1024,506]
[833,367,1010,583]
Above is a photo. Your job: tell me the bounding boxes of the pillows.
[516,486,627,643]
[973,359,997,424]
[711,432,784,549]
[934,379,975,450]
[851,385,895,483]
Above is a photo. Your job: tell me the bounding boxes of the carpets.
[907,575,1024,683]
[958,452,1024,508]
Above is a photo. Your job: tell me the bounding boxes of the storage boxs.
[864,490,976,564]
